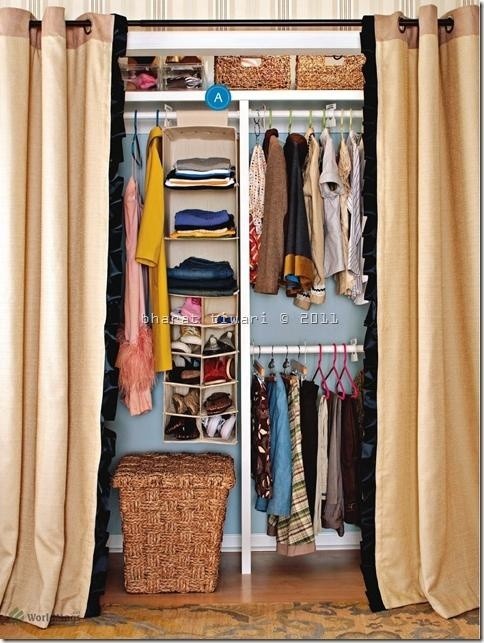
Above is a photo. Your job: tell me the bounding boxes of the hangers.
[253,344,359,400]
[130,109,161,169]
[261,105,363,135]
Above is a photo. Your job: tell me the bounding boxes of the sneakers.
[165,326,237,441]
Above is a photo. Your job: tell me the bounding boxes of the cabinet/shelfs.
[115,31,370,578]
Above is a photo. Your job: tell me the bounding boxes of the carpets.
[2,601,480,641]
